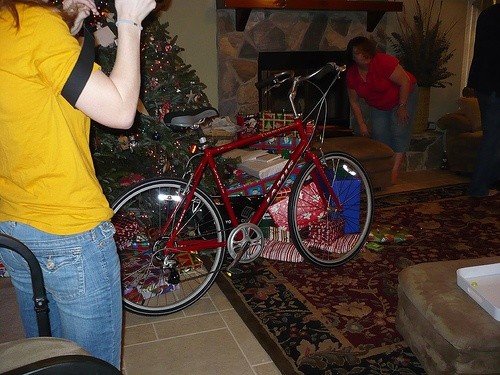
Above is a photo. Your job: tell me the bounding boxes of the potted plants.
[382,0,459,132]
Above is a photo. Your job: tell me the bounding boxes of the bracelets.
[399,103,408,106]
[115,19,143,32]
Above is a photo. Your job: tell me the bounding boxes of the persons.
[0,0,157,372]
[462,2,500,195]
[345,37,418,184]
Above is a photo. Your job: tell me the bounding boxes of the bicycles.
[108,59,375,316]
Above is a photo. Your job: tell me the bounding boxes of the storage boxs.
[221,112,361,243]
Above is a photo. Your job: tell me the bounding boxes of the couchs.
[439,100,484,171]
[394,257,500,375]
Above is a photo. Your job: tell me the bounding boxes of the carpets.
[204,183,500,375]
